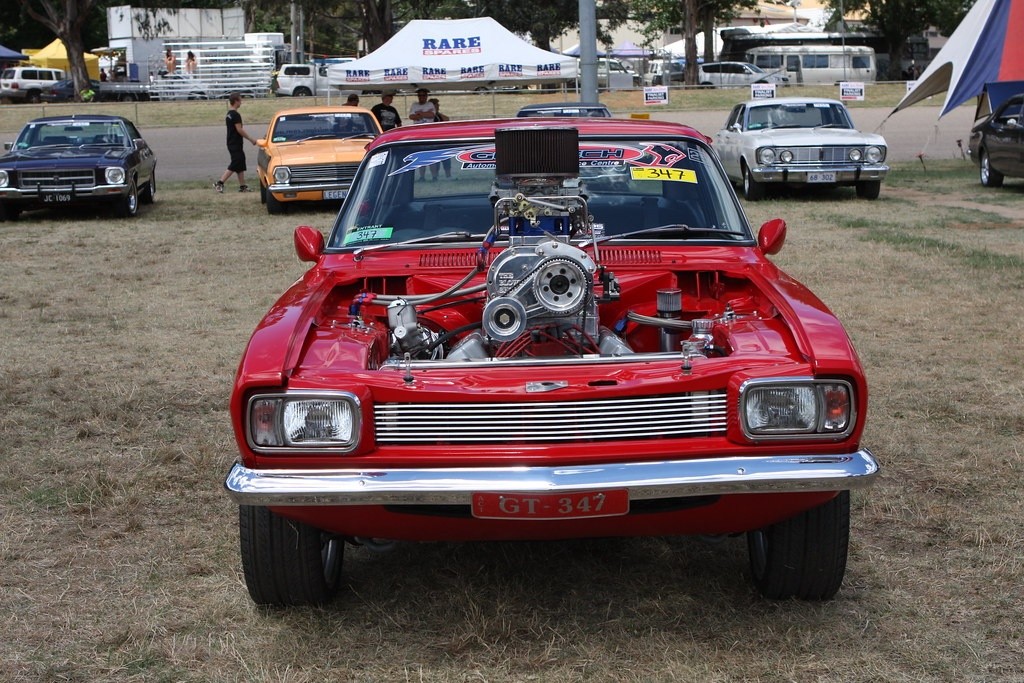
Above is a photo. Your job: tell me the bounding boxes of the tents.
[30,38,99,82]
[609,40,657,81]
[0,45,29,67]
[657,21,826,86]
[563,43,608,88]
[873,0,1024,176]
[325,17,579,127]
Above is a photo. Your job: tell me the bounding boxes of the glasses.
[418,94,427,97]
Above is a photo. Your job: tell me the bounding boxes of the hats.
[415,87,430,93]
[381,91,397,97]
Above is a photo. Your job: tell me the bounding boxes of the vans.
[745,44,875,88]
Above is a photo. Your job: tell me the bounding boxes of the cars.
[226,116,881,601]
[1,114,158,214]
[567,58,644,90]
[258,106,385,213]
[711,97,888,199]
[967,92,1024,188]
[273,63,364,96]
[639,60,686,87]
[697,61,783,92]
[0,66,68,103]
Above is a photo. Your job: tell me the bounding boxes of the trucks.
[100,70,210,100]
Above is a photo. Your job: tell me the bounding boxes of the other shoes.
[239,185,253,192]
[212,181,224,193]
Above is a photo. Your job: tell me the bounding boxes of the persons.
[340,94,359,107]
[184,51,198,75]
[212,92,258,193]
[371,87,402,131]
[164,47,177,75]
[99,68,108,81]
[80,84,96,102]
[408,88,453,182]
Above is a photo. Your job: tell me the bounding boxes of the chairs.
[92,134,119,144]
[43,136,69,145]
[422,202,494,231]
[589,197,659,235]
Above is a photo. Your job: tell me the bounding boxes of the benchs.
[68,136,128,144]
[148,41,276,101]
[383,197,697,234]
